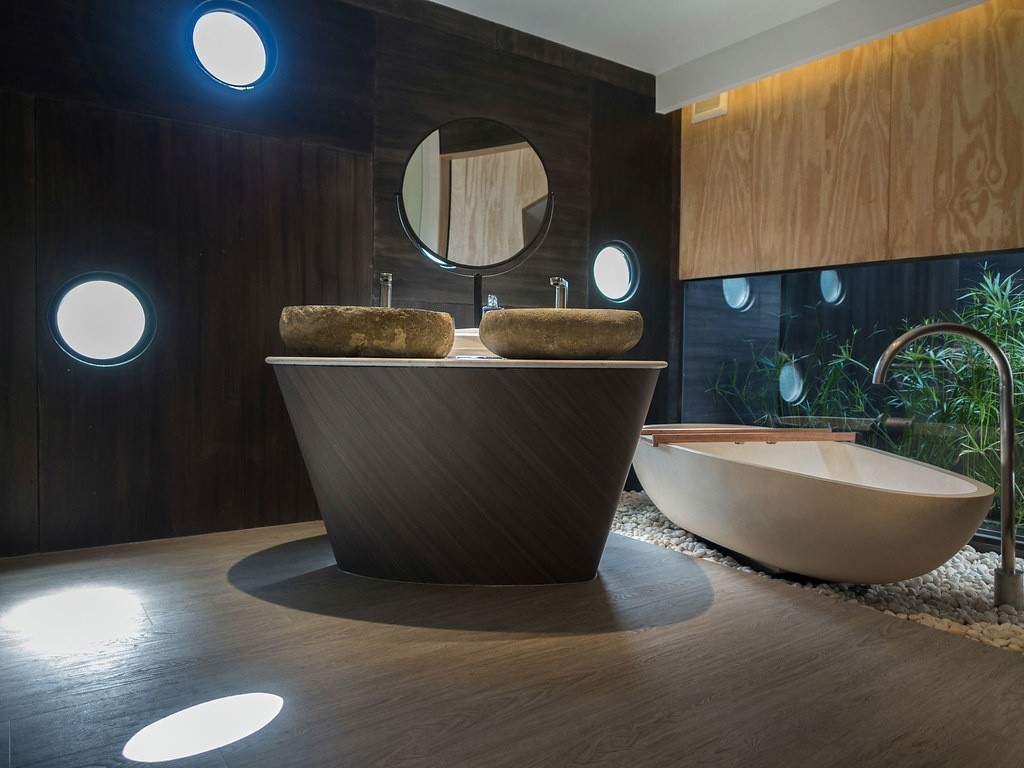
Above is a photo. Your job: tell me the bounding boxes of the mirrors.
[394,116,555,326]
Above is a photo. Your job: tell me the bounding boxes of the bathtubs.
[633,420,997,588]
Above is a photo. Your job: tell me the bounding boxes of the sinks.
[477,306,644,358]
[276,304,456,358]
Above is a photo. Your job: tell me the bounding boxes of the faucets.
[548,274,570,307]
[376,270,393,309]
[869,322,1024,611]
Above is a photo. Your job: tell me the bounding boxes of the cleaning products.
[481,293,504,317]
